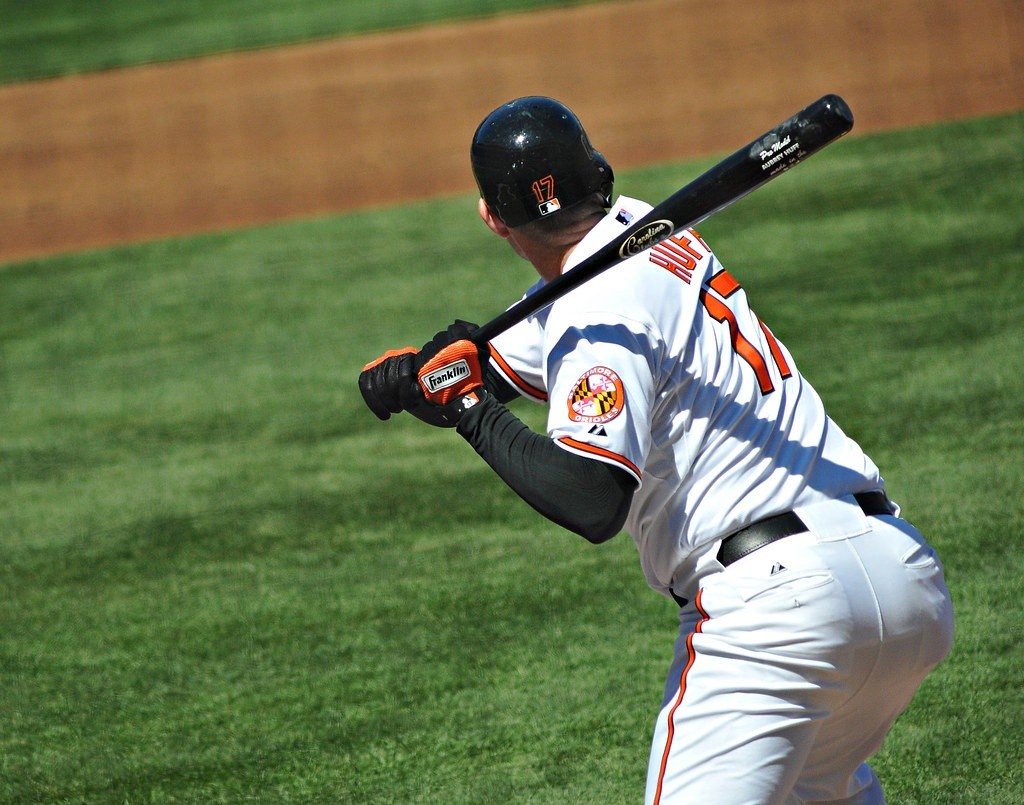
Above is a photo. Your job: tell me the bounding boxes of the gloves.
[410,318,487,425]
[358,346,454,428]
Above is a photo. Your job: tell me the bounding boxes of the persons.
[358,98,955,805]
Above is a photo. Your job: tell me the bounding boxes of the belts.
[669,492,893,608]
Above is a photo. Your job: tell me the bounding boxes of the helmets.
[470,95,615,228]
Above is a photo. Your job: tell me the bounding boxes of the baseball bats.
[470,91,855,350]
